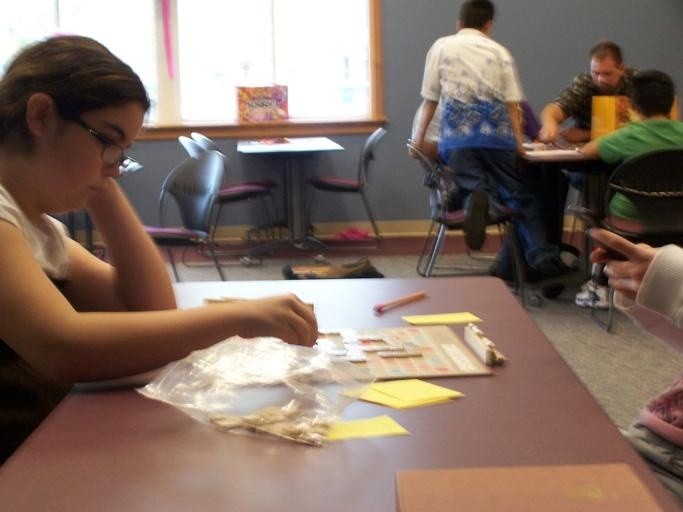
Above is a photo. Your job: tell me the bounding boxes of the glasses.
[47,91,144,177]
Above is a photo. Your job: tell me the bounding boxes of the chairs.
[303,127,389,252]
[112,151,230,280]
[404,123,683,334]
[178,135,278,258]
[191,129,282,253]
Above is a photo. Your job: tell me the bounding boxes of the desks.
[1,273,683,511]
[235,134,349,267]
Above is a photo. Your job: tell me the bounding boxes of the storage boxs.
[590,95,679,145]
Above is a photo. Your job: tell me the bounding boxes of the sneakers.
[574,278,611,310]
[463,189,490,252]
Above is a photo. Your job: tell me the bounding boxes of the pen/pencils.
[375,292,426,314]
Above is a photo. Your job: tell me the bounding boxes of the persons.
[0,37,317,384]
[409,0,567,278]
[574,70,683,305]
[539,40,650,185]
[415,94,520,280]
[587,222,683,512]
[518,101,567,275]
